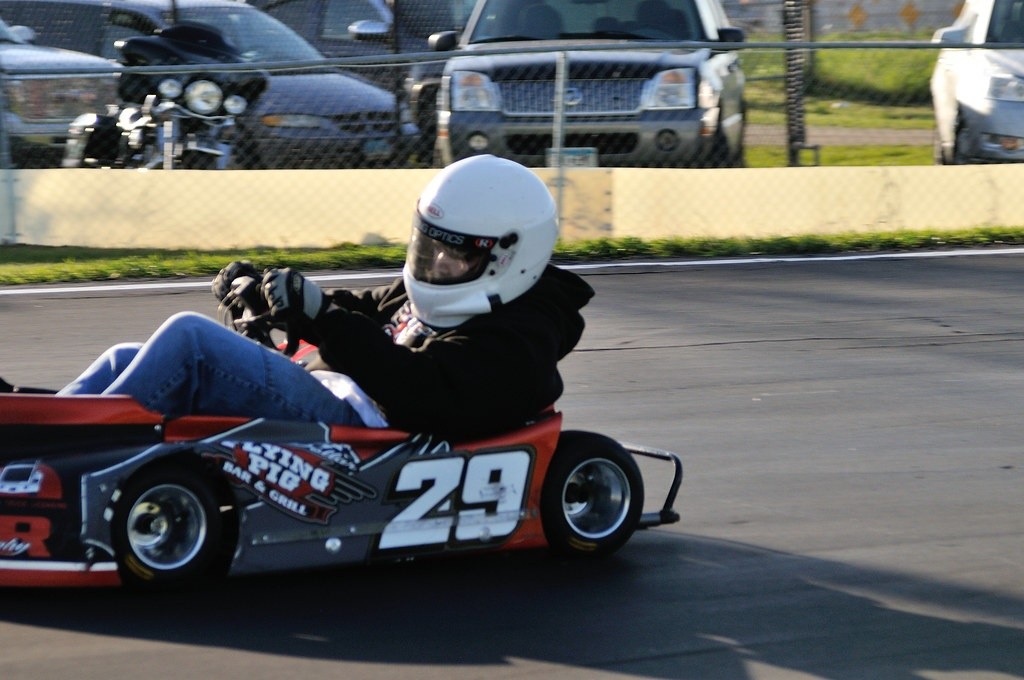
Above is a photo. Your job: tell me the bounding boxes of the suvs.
[427,0,751,167]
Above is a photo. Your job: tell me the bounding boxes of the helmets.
[402,155,556,330]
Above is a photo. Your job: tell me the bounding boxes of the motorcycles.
[13,0,268,171]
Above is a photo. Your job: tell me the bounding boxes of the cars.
[929,0,1024,165]
[0,0,423,168]
[245,0,452,156]
[1,18,126,169]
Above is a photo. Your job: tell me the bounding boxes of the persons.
[53,153,597,443]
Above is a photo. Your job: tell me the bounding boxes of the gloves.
[210,259,255,302]
[261,266,332,328]
